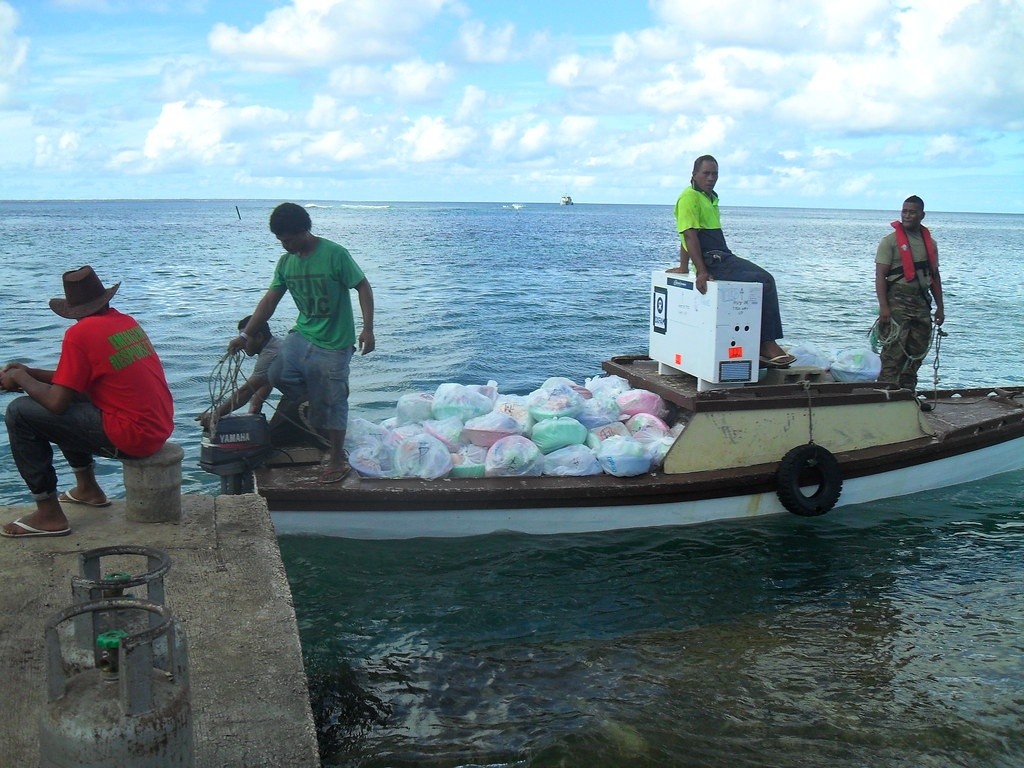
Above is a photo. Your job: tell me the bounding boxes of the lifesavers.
[773,442,844,517]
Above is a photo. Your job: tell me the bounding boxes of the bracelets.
[239,331,248,340]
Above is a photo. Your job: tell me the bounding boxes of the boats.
[195,369,1024,541]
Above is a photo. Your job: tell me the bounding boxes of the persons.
[194,314,350,444]
[874,195,944,413]
[665,154,798,366]
[227,204,376,485]
[0,265,175,540]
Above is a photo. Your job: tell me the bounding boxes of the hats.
[49,265,121,318]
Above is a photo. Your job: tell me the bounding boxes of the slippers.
[57,488,111,507]
[318,464,352,484]
[0,517,72,538]
[760,347,796,366]
